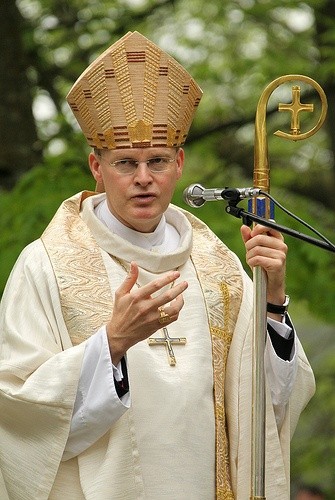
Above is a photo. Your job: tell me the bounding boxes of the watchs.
[267,294,290,315]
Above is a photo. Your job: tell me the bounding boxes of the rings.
[157,306,169,325]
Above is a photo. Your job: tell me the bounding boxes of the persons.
[1,30,317,500]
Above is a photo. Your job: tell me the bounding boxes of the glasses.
[96,153,177,175]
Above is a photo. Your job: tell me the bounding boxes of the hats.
[65,30,203,150]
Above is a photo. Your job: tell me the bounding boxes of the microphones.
[183,184,261,208]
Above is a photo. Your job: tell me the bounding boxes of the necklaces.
[112,257,187,366]
